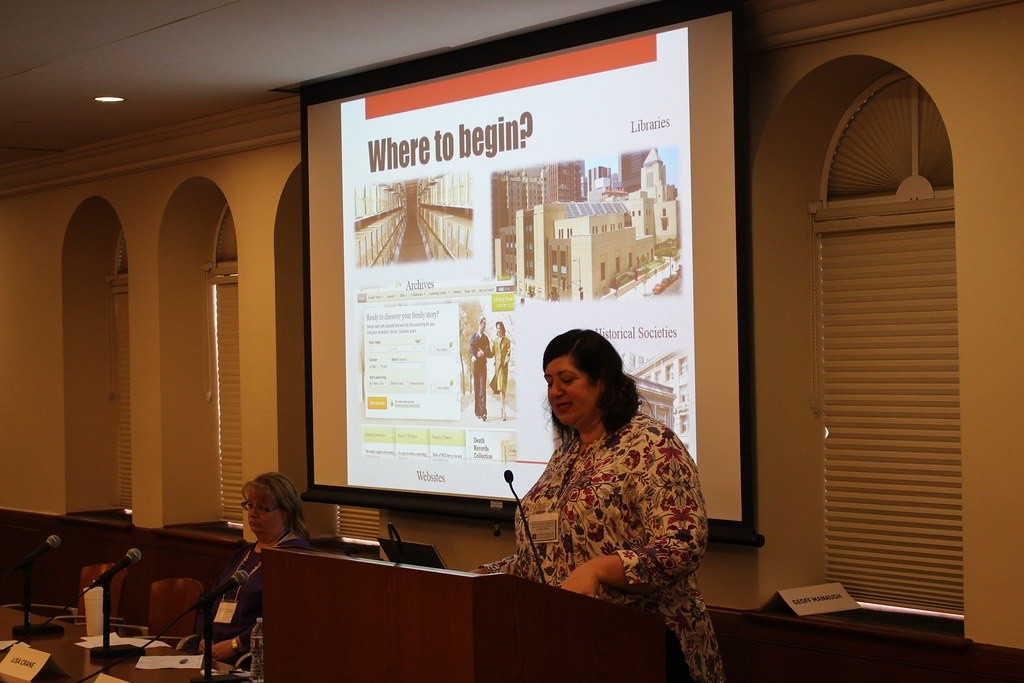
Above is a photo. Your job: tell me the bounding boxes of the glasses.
[240,500,279,514]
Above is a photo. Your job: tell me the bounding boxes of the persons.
[196,471,313,671]
[470,315,511,422]
[472,329,727,683]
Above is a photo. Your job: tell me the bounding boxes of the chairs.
[78,564,131,622]
[146,578,204,638]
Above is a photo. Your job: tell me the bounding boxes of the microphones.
[10,535,60,575]
[188,570,249,612]
[504,470,546,584]
[90,548,142,589]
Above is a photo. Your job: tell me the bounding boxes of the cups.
[83,587,103,636]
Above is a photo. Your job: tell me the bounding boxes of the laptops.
[377,537,447,569]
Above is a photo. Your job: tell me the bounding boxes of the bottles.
[250,617,264,683]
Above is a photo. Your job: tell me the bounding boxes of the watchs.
[231,637,241,654]
